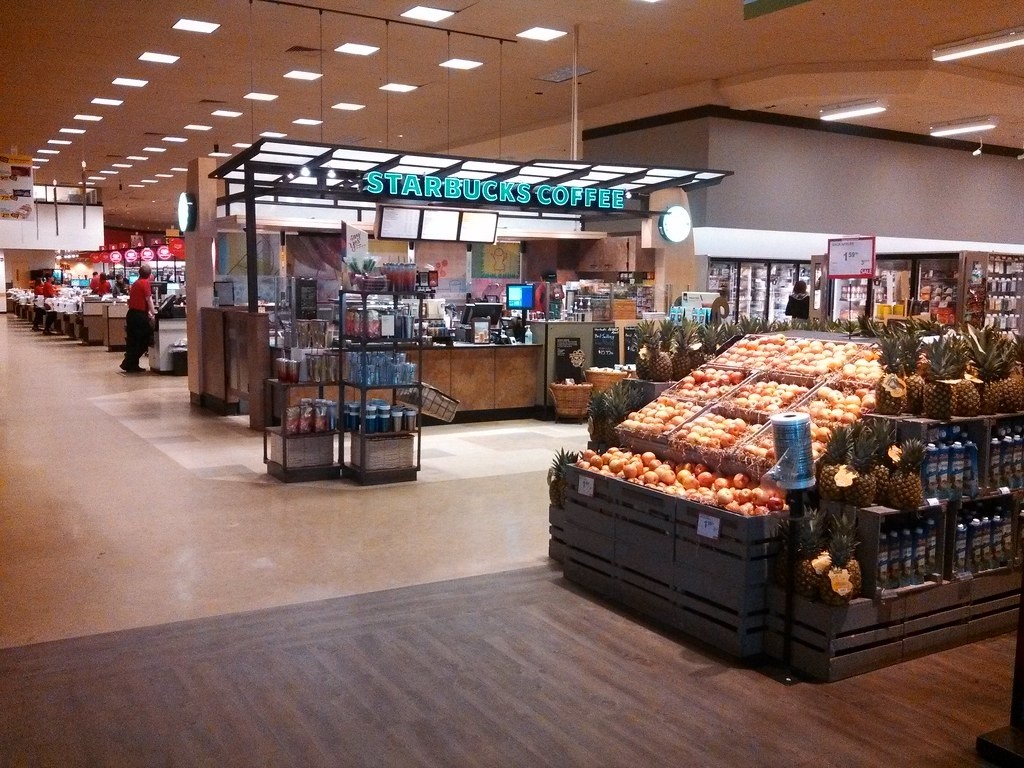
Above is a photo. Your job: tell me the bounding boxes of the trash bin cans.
[169,341,189,377]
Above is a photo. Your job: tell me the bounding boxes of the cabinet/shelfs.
[263,221,436,487]
[578,236,654,272]
[580,282,656,321]
[826,252,988,328]
[520,319,646,408]
[271,347,536,420]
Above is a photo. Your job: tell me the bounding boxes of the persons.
[32,278,44,331]
[119,264,158,372]
[785,281,809,319]
[90,272,111,296]
[43,274,63,335]
[112,275,129,298]
[536,271,564,311]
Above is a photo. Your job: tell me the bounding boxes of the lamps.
[658,205,691,243]
[177,192,196,231]
[820,25,1024,138]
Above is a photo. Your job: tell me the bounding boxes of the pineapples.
[794,326,1024,604]
[549,314,848,509]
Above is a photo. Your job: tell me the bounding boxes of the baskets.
[550,381,594,419]
[585,369,628,403]
[622,368,637,379]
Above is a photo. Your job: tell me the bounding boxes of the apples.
[576,332,885,516]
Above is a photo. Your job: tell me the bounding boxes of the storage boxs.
[350,431,415,470]
[395,381,460,422]
[270,432,334,468]
[548,331,1024,686]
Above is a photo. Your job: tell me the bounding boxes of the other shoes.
[31,327,42,332]
[43,330,55,335]
[120,363,146,373]
